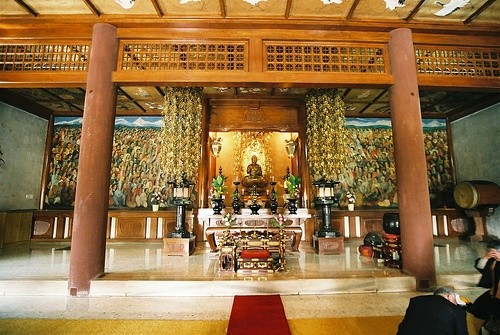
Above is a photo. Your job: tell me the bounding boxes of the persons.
[241,154,268,188]
[455,259,500,335]
[474,239,500,289]
[396,286,469,335]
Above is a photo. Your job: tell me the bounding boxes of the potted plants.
[209,176,227,215]
[285,175,300,215]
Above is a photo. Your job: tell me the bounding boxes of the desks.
[205,226,303,252]
